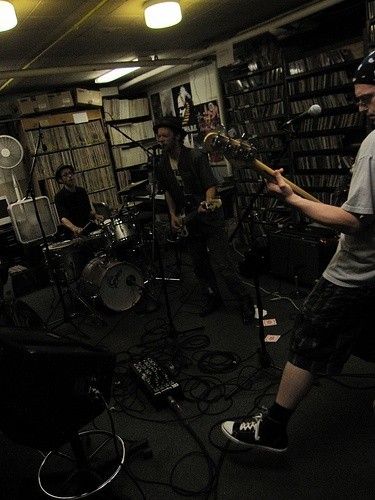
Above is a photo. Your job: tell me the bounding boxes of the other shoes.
[239,296,255,324]
[199,296,223,317]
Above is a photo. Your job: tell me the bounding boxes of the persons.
[153,115,255,325]
[220,46,375,463]
[54,163,105,277]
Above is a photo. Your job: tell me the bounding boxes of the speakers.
[0,326,113,441]
[265,233,338,290]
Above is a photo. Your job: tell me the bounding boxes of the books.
[26,97,158,228]
[224,43,367,237]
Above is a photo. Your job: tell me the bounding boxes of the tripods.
[25,126,322,402]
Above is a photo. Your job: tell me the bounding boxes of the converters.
[129,358,183,411]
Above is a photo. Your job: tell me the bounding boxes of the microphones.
[280,104,322,126]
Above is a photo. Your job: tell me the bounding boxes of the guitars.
[203,130,324,204]
[165,195,222,243]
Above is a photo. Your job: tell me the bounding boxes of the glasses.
[355,92,375,106]
[59,172,74,178]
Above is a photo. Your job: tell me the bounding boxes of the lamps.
[142,0,182,29]
[95,57,152,83]
[0,0,18,32]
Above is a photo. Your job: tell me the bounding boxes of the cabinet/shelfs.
[102,92,159,205]
[220,0,375,248]
[0,226,51,298]
[16,105,122,226]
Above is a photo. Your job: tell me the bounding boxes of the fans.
[0,135,25,215]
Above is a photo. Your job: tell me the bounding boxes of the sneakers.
[221,406,289,453]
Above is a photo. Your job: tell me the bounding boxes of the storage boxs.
[16,87,119,116]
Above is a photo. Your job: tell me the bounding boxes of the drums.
[99,216,137,250]
[76,228,107,255]
[41,239,84,286]
[80,253,144,312]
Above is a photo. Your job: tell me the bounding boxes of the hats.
[352,50,375,85]
[55,164,74,184]
[153,116,186,139]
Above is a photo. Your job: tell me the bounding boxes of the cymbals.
[112,201,143,209]
[135,194,165,200]
[117,178,149,195]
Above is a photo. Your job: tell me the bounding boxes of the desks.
[127,185,236,240]
